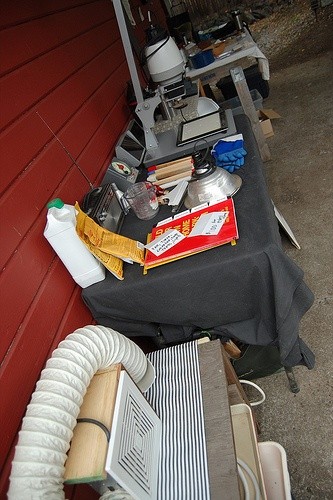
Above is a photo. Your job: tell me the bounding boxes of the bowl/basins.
[188,49,215,69]
[202,41,225,56]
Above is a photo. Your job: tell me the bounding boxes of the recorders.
[85,185,126,236]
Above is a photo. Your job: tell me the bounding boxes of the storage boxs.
[256,108,282,140]
[190,48,215,68]
[216,88,265,117]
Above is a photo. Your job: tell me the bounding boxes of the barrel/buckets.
[43,198,107,289]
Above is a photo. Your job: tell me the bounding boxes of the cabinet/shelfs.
[133,335,268,500]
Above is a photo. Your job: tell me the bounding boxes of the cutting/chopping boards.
[62,363,121,483]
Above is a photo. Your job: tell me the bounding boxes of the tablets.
[176,108,228,146]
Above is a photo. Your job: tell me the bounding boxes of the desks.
[181,25,271,101]
[78,112,316,401]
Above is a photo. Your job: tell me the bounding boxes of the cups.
[120,180,160,221]
[126,182,147,202]
